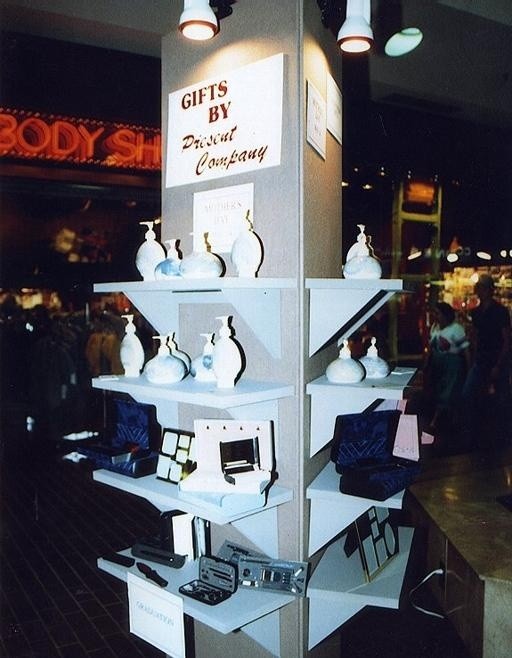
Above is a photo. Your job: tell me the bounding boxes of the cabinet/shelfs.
[94,374,295,459]
[305,460,406,559]
[306,367,421,457]
[306,527,416,651]
[306,275,416,358]
[94,468,294,559]
[97,546,295,658]
[95,277,295,360]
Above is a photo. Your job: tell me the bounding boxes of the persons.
[472,273,512,380]
[426,302,470,430]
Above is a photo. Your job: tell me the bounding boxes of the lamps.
[336,0,373,51]
[407,249,420,259]
[178,0,217,40]
